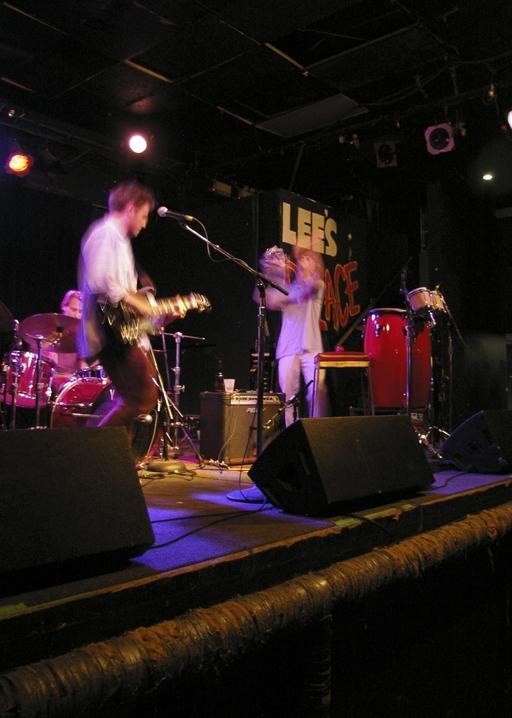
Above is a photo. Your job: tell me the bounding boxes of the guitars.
[100,293,211,343]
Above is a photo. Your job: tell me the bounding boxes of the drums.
[364,307,431,410]
[426,290,448,323]
[408,287,429,319]
[53,376,162,469]
[2,350,51,409]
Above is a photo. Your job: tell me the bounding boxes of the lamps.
[128,132,148,156]
[478,157,497,185]
[3,136,36,179]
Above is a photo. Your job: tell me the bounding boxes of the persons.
[253,246,326,425]
[80,181,157,428]
[41,290,100,371]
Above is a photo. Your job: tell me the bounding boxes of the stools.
[310,350,374,418]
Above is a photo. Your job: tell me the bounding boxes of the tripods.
[402,322,445,461]
[147,338,201,467]
[423,325,451,447]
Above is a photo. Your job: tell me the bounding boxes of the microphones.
[156,205,196,225]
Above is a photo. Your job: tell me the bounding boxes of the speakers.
[247,415,435,515]
[440,409,511,473]
[0,426,156,591]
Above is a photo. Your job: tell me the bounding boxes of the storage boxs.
[198,390,283,464]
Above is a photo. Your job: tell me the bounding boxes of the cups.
[224,378,235,393]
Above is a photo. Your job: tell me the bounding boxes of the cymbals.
[17,314,83,352]
